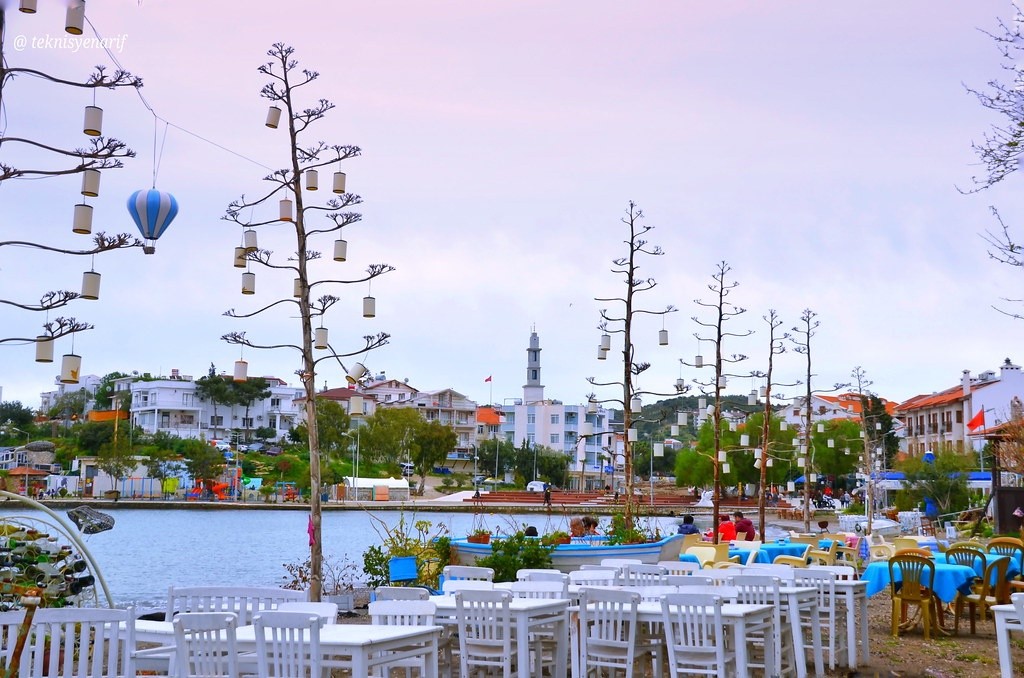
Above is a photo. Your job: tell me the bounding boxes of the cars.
[238,443,284,456]
[470,476,486,485]
[210,439,230,453]
[527,481,545,492]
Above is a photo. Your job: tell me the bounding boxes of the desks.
[595,582,822,678]
[975,543,1023,576]
[929,553,1016,587]
[857,563,975,637]
[182,624,443,678]
[919,540,947,552]
[760,541,810,562]
[88,615,190,678]
[727,550,774,566]
[411,595,569,677]
[990,604,1024,678]
[569,603,779,678]
[780,579,870,672]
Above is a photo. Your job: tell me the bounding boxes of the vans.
[399,463,415,475]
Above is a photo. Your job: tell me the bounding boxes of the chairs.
[0,526,1024,678]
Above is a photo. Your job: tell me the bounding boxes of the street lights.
[341,432,355,500]
[12,427,30,497]
[225,428,239,503]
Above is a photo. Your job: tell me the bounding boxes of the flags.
[967,409,984,431]
[485,376,491,382]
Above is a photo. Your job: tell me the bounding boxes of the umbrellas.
[922,450,938,517]
[794,477,804,483]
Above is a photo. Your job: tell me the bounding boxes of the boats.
[430,533,684,572]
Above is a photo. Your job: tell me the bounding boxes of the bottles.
[0,523,97,662]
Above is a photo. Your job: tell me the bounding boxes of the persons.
[39,487,44,500]
[814,488,850,509]
[614,490,618,505]
[766,492,778,507]
[543,489,550,504]
[733,511,755,541]
[1013,506,1024,530]
[570,517,599,537]
[525,526,538,537]
[706,513,736,541]
[605,484,610,494]
[677,516,699,533]
